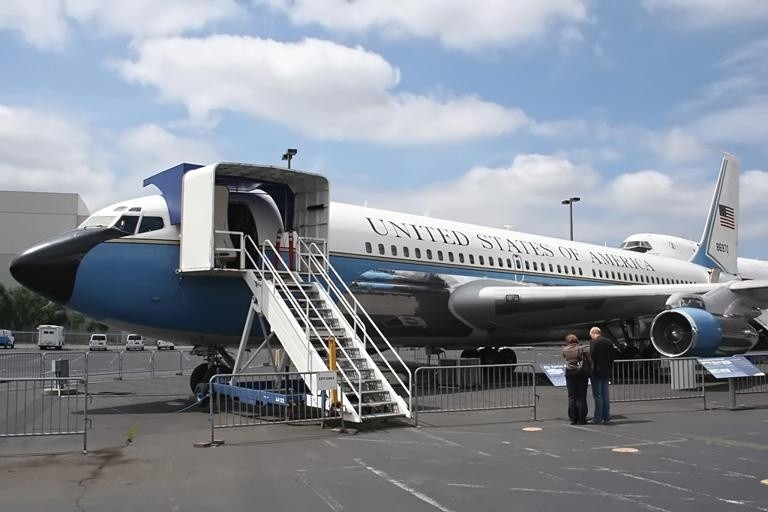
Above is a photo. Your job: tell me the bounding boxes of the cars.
[156,340,175,351]
[0,328,17,349]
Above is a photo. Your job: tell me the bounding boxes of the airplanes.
[7,148,768,404]
[615,231,767,330]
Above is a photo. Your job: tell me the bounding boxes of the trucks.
[36,325,65,350]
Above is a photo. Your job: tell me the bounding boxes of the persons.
[562,333,595,425]
[587,325,616,425]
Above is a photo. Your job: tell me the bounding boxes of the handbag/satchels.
[575,359,591,377]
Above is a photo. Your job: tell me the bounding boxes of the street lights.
[281,149,298,171]
[561,196,582,240]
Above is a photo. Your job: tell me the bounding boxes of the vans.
[86,333,108,351]
[124,334,145,352]
